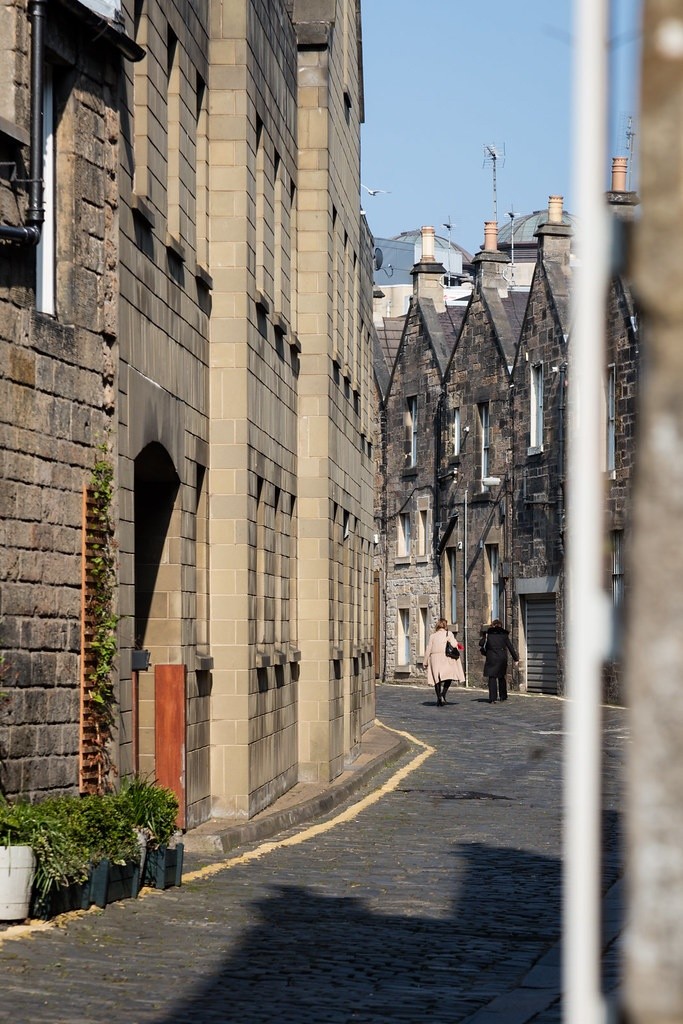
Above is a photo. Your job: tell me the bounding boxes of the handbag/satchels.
[480,646,486,656]
[446,642,460,660]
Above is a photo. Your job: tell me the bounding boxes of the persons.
[479,619,521,703]
[423,619,463,708]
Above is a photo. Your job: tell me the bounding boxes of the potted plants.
[0,765,186,922]
